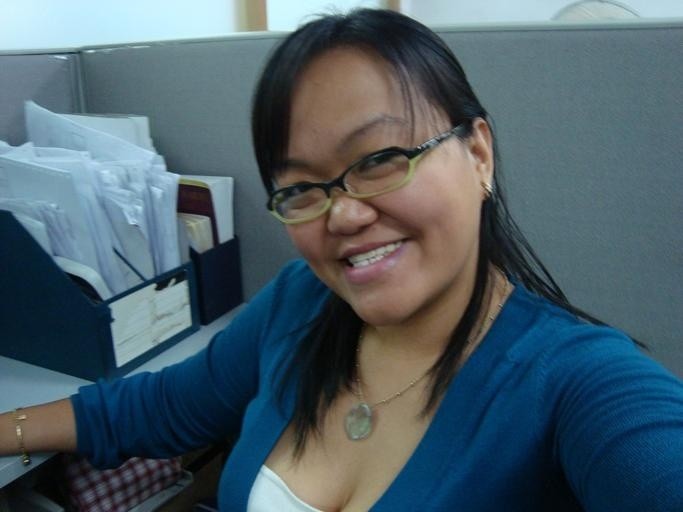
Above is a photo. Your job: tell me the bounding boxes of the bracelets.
[13,406,32,467]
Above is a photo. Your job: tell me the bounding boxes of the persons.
[0,9,681,511]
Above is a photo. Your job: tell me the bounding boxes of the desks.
[0,301,259,487]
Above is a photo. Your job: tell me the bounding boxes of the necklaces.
[342,319,442,442]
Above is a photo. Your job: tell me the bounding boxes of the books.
[178,174,237,253]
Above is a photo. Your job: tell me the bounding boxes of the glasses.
[266,122,475,225]
